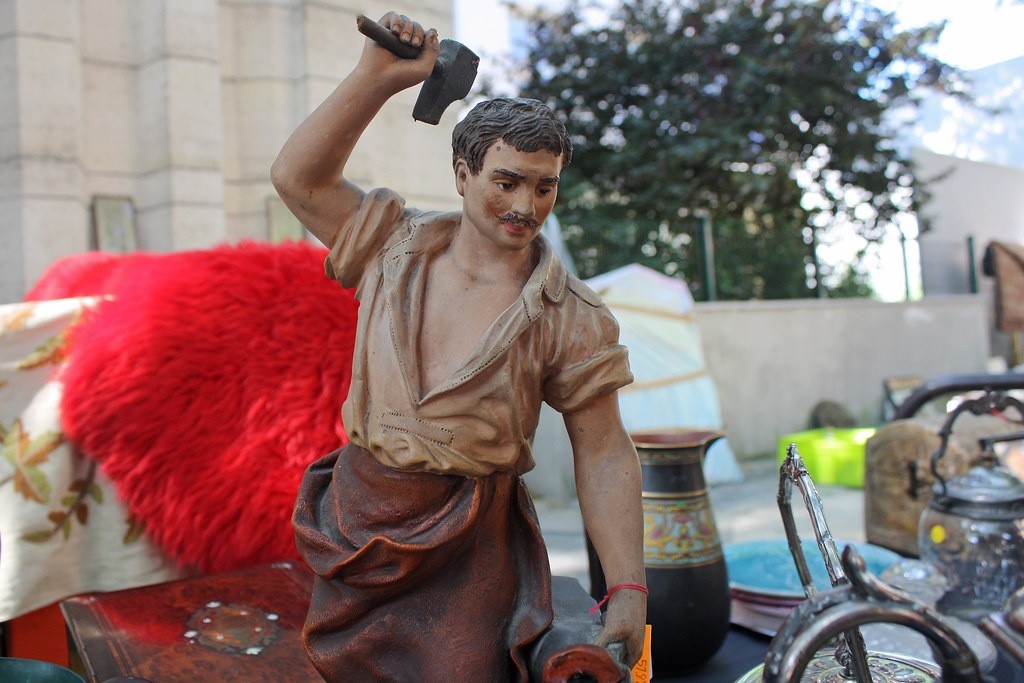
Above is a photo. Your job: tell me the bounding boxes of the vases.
[591,430,732,678]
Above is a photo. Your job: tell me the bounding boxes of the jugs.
[584,430,728,675]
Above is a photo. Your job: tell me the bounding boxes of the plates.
[719,537,903,609]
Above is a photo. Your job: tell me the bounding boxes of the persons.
[268,10,646,683]
